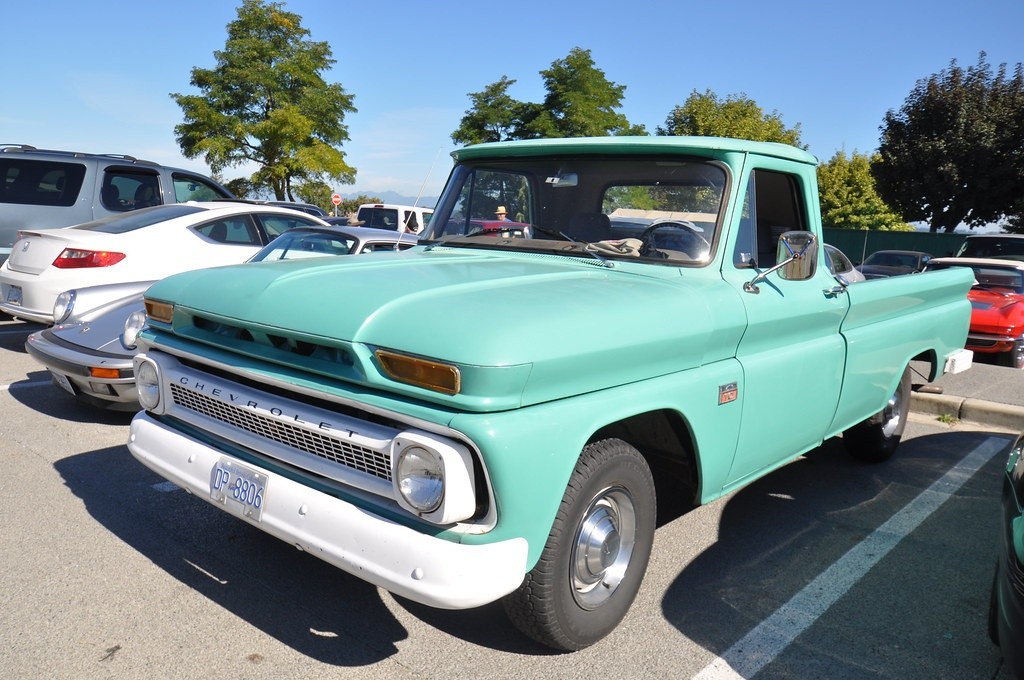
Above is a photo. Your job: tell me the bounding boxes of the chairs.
[208,222,228,242]
[108,184,123,211]
[133,183,152,206]
[56,176,64,190]
[569,213,612,242]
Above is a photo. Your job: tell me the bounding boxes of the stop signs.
[332,195,341,205]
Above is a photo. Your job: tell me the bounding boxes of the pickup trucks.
[128,134,977,654]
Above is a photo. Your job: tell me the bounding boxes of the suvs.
[0,143,272,266]
[950,235,1024,288]
[356,204,436,235]
[211,198,348,251]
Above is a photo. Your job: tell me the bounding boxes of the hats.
[494,206,508,214]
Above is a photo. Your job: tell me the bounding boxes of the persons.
[494,206,512,222]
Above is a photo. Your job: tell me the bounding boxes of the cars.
[445,220,531,239]
[855,250,932,280]
[0,205,373,327]
[988,428,1023,680]
[911,256,1024,368]
[822,243,866,284]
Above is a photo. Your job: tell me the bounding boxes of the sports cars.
[22,226,422,415]
